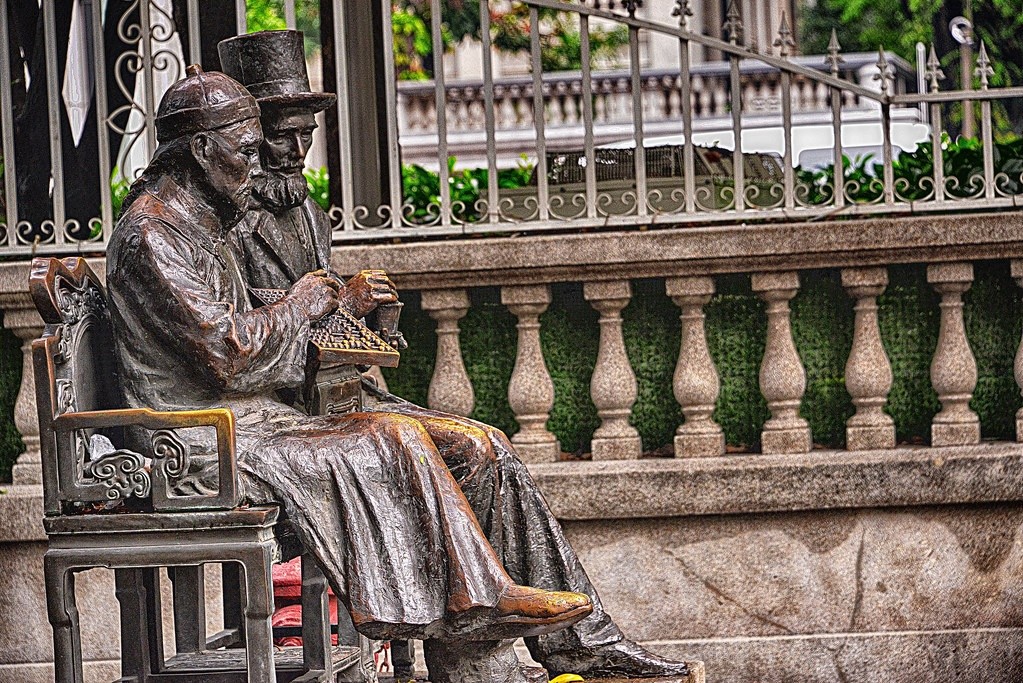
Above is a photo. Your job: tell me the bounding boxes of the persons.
[105,63,594,683]
[217,30,687,681]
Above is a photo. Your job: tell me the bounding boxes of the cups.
[365,303,404,337]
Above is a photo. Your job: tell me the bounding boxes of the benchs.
[27,256,416,683]
